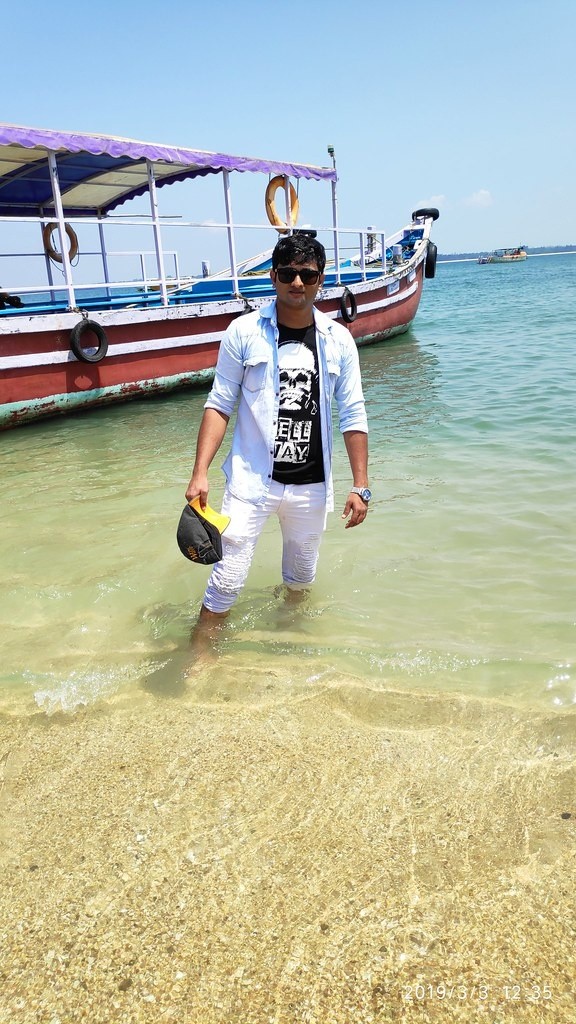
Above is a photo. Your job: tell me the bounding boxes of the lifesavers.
[424,243,438,280]
[43,220,78,262]
[340,290,358,323]
[69,319,108,363]
[410,208,442,222]
[265,174,301,235]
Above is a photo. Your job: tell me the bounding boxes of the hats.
[177,494,231,565]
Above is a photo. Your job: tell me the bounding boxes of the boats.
[0,122,440,433]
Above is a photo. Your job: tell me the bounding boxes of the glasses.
[275,267,321,285]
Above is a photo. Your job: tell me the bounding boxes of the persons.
[180,231,374,654]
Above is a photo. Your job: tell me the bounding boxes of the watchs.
[350,486,373,503]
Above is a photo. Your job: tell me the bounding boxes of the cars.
[477,244,528,265]
